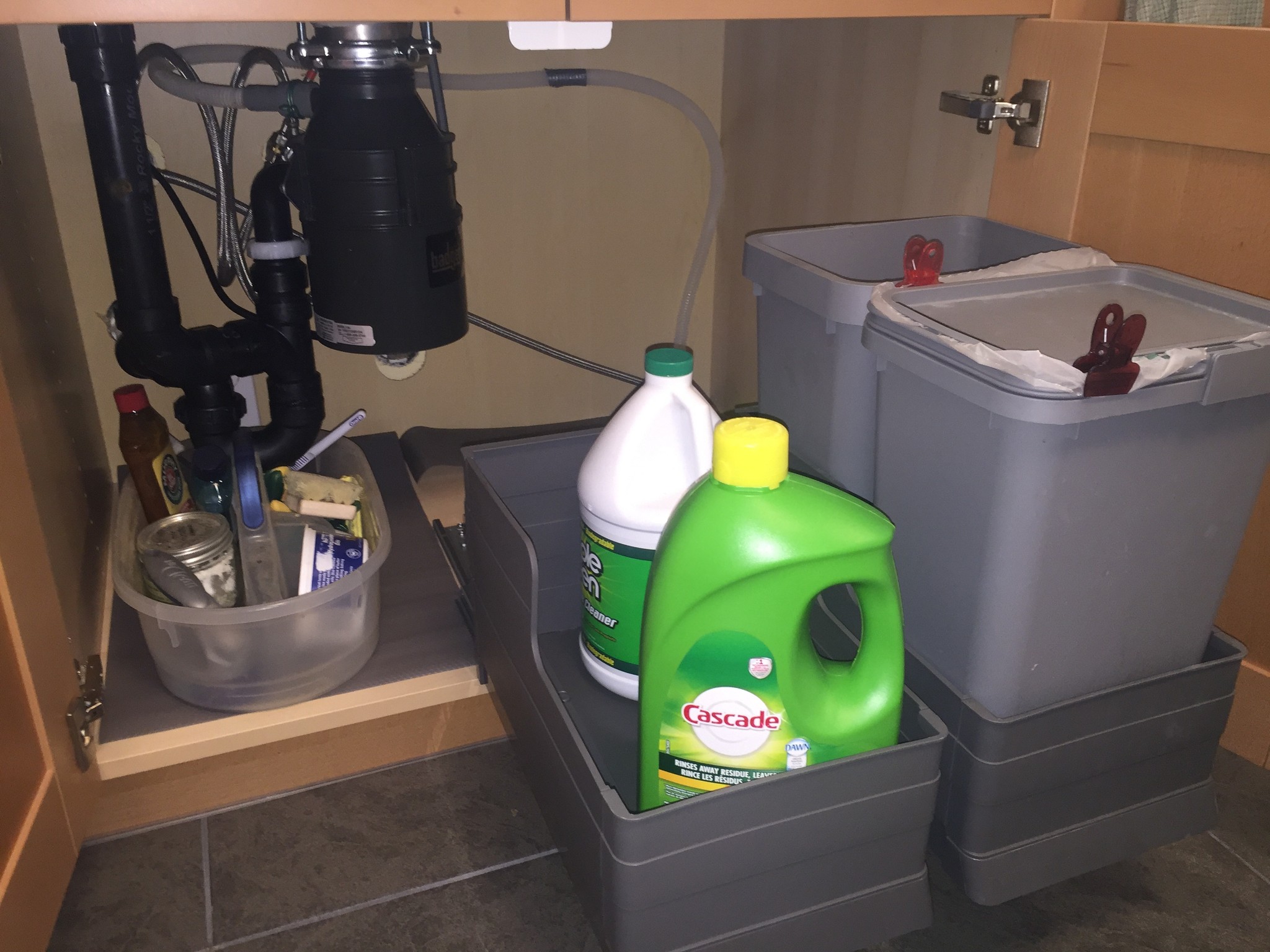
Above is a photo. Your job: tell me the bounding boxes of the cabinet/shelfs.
[0,0,1270,947]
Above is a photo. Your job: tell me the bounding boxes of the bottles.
[113,383,197,525]
[637,418,904,815]
[577,347,724,702]
[135,510,239,611]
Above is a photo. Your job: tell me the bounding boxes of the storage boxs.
[741,215,1077,497]
[109,423,392,717]
[860,260,1270,728]
[453,426,955,949]
[892,622,1251,909]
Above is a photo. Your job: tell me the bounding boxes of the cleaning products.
[641,415,906,819]
[110,383,195,525]
[575,344,723,697]
